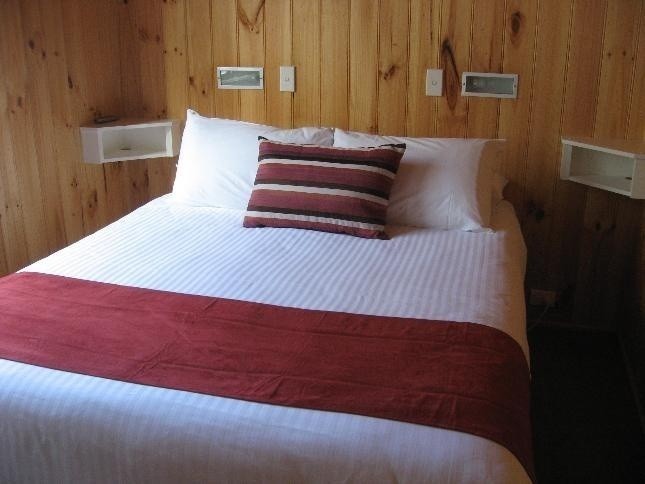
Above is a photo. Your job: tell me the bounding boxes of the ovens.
[1,183,530,483]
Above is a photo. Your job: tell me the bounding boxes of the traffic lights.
[241,133,408,240]
[330,122,508,236]
[172,106,334,209]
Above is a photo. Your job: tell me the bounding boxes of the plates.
[76,112,183,166]
[558,134,645,201]
